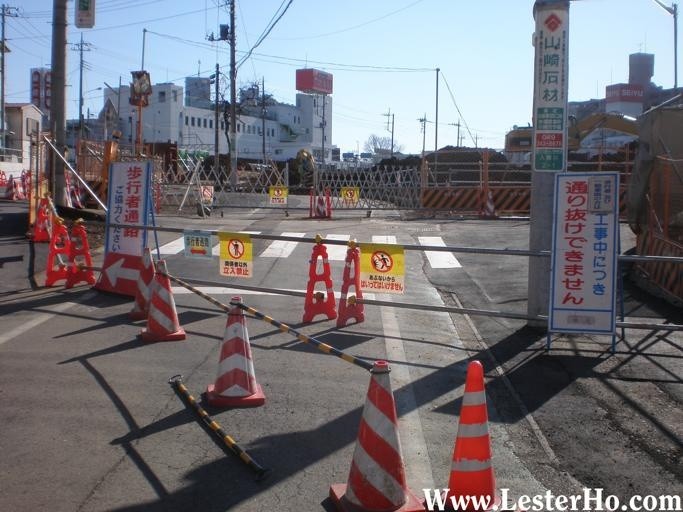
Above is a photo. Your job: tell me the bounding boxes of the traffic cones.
[6,181,16,202]
[329,361,425,512]
[435,360,502,511]
[127,248,185,341]
[206,296,266,406]
